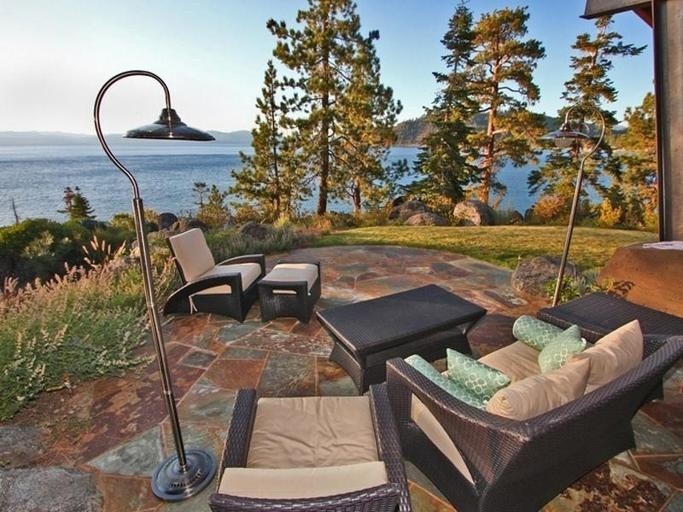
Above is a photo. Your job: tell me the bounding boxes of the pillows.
[538,322,587,373]
[512,313,595,352]
[403,353,487,410]
[446,347,511,404]
[561,317,644,396]
[484,357,592,422]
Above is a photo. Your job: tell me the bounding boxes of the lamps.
[535,104,605,306]
[92,69,217,504]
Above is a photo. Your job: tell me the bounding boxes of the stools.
[255,259,322,325]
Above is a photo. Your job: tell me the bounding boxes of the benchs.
[535,291,683,402]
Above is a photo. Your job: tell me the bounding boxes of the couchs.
[162,227,267,325]
[206,382,412,511]
[385,307,683,511]
